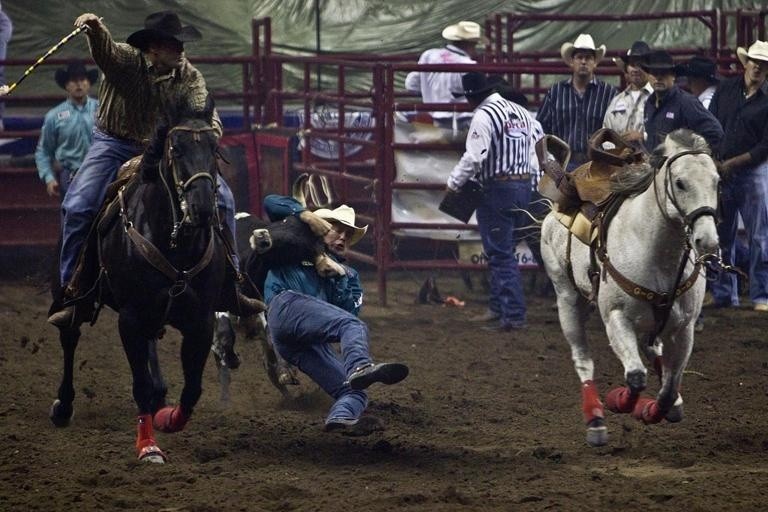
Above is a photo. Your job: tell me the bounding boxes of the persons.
[535,33,622,177]
[0,8,13,137]
[686,58,718,112]
[262,193,410,428]
[46,10,266,328]
[438,70,547,331]
[405,19,482,138]
[708,39,768,311]
[34,61,99,318]
[603,41,656,135]
[639,49,725,160]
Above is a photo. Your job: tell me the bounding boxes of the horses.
[51,92,217,462]
[510,128,726,447]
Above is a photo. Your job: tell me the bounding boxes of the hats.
[680,54,720,82]
[559,33,607,68]
[314,205,367,246]
[619,40,648,64]
[55,57,100,91]
[735,40,767,69]
[440,20,486,43]
[124,11,204,47]
[638,50,685,75]
[450,72,506,99]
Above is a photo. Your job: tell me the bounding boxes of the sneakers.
[346,361,411,388]
[329,417,364,435]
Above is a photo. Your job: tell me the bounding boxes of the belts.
[487,175,534,181]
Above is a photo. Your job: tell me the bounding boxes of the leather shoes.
[702,299,719,309]
[469,310,497,320]
[754,301,765,312]
[48,294,96,325]
[232,288,269,317]
